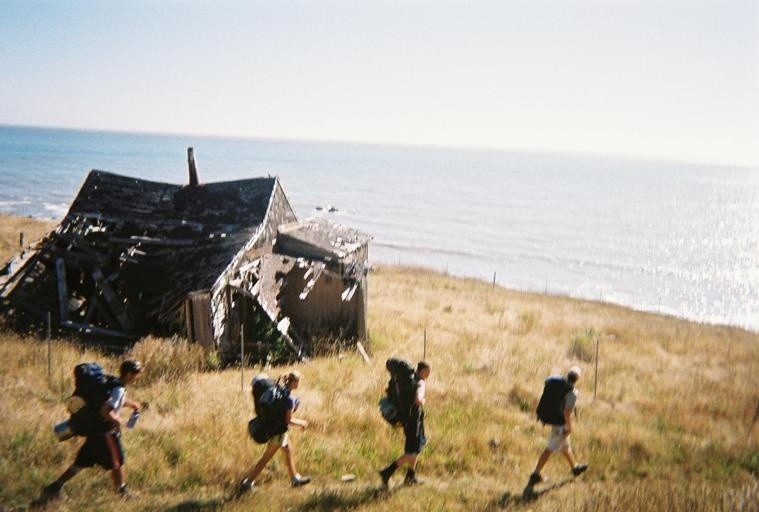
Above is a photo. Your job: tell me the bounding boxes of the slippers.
[378,357,424,426]
[248,374,291,443]
[54,361,124,443]
[535,374,574,426]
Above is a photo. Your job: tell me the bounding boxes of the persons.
[43,358,142,497]
[529,370,587,482]
[239,373,312,490]
[378,362,431,486]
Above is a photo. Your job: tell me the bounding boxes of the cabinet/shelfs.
[420,436,427,446]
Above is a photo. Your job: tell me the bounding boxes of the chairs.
[404,477,424,486]
[292,477,310,487]
[531,475,541,482]
[573,464,588,476]
[380,470,390,485]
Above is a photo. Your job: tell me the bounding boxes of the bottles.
[567,366,581,378]
